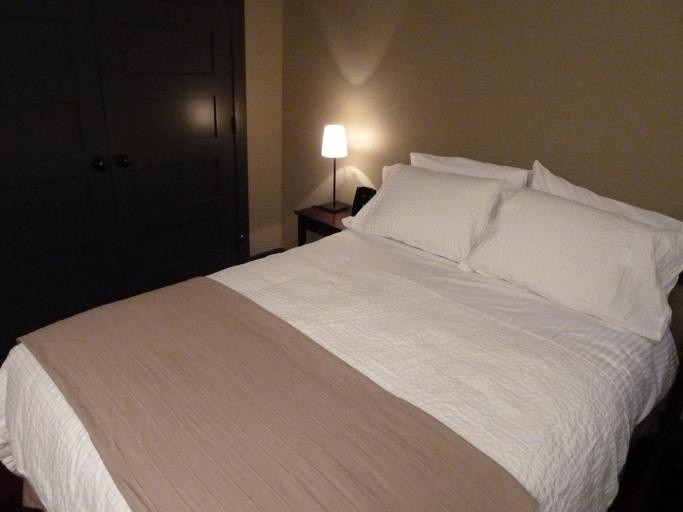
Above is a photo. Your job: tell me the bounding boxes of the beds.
[0,152,683,511]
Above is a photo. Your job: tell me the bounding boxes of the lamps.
[314,124,353,213]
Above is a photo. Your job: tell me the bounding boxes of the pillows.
[341,163,519,263]
[410,152,532,187]
[457,186,683,343]
[527,158,680,232]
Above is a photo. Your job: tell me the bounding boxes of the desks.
[294,205,354,246]
